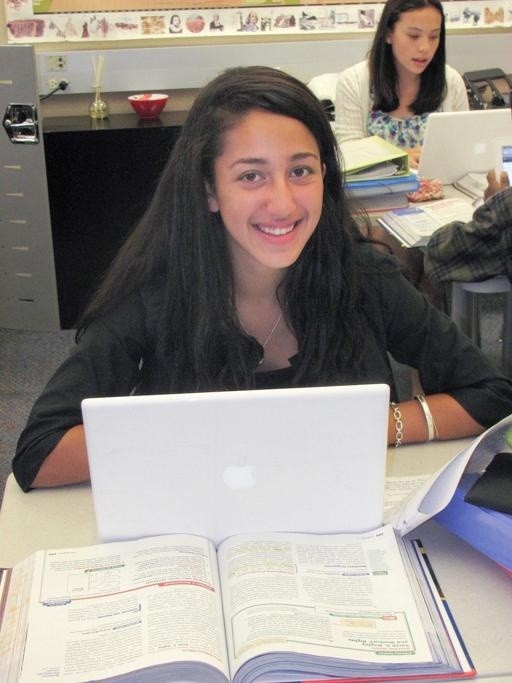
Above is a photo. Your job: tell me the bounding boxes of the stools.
[447,274,511,374]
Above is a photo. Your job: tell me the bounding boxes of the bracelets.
[388,401,404,447]
[414,393,439,441]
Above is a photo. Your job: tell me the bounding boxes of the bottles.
[88,84,110,120]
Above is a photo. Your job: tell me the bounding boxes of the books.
[0,526,476,680]
[329,134,501,247]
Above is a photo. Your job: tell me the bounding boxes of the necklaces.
[231,305,283,365]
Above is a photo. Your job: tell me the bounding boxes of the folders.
[338,134,410,183]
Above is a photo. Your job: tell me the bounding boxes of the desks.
[0,417,511,682]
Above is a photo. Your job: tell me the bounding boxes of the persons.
[168,14,183,33]
[332,0,470,166]
[422,167,511,290]
[11,65,512,494]
[243,10,258,32]
[210,14,224,32]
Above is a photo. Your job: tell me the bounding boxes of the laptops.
[81,384,390,552]
[409,108,512,186]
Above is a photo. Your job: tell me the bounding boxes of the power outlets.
[43,55,67,74]
[48,78,71,92]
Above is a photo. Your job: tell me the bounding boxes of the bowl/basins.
[127,93,169,119]
